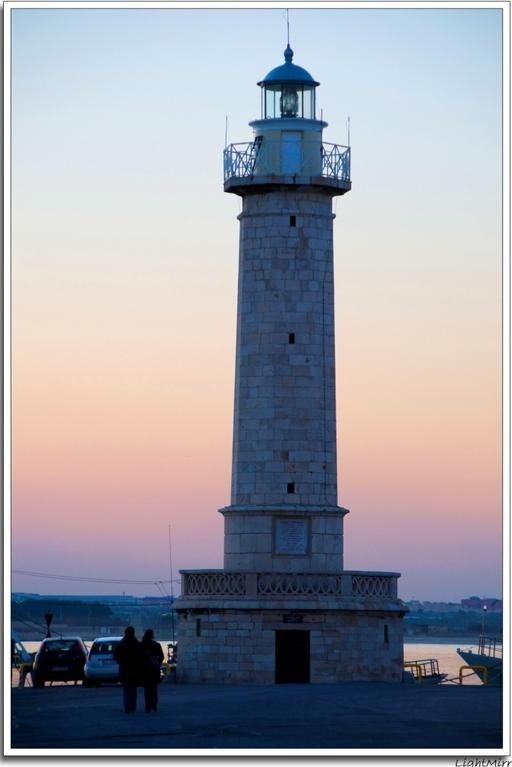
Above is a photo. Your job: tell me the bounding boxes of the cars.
[31,635,89,688]
[81,635,124,686]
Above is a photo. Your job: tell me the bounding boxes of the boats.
[405,659,449,685]
[454,604,503,684]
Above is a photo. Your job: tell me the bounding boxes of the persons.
[138,629,164,713]
[114,626,141,713]
